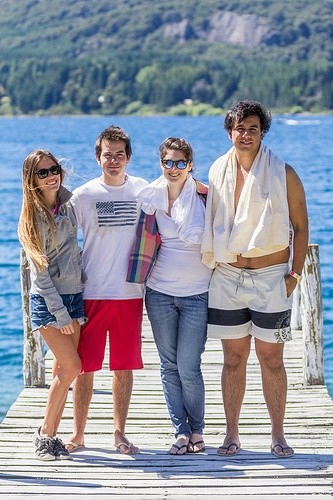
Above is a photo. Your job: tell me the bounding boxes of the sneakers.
[33,426,55,460]
[53,436,73,459]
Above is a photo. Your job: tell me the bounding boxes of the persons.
[18,149,87,460]
[63,125,150,454]
[127,137,213,456]
[200,100,309,458]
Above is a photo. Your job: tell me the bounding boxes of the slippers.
[187,439,205,453]
[270,443,295,458]
[65,442,85,453]
[113,442,141,454]
[168,444,188,455]
[217,443,240,457]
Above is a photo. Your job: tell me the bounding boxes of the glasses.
[36,165,62,179]
[162,160,191,169]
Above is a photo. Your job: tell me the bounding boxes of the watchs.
[288,271,303,283]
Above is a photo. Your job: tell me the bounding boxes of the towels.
[138,171,205,244]
[200,141,289,269]
